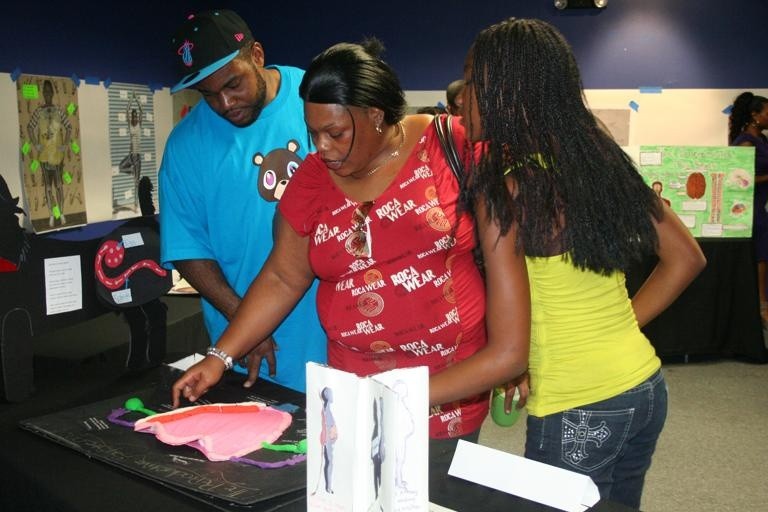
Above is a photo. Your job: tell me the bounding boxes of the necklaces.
[352,121,406,180]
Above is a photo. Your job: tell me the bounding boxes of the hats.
[158,6,253,95]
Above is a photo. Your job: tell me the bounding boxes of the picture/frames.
[640,145,756,240]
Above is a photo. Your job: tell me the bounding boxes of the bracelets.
[205,346,235,371]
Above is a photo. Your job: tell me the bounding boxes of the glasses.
[349,199,375,259]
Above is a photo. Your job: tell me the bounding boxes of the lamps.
[553,0,608,9]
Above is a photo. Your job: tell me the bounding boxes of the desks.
[0,351,640,512]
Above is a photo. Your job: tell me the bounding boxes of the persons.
[27,80,73,229]
[172,41,530,445]
[118,90,144,215]
[429,18,708,509]
[441,80,465,116]
[728,92,768,340]
[158,10,331,397]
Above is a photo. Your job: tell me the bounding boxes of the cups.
[490,384,526,426]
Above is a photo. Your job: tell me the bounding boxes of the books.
[306,361,430,512]
